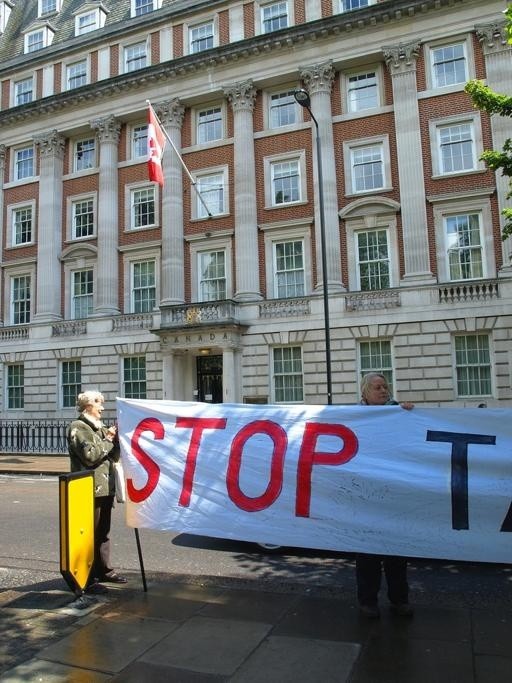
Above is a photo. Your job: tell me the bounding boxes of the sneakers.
[359,605,381,619]
[388,601,414,616]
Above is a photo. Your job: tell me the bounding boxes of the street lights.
[292,89,331,405]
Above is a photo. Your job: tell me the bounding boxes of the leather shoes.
[86,583,109,594]
[102,571,127,582]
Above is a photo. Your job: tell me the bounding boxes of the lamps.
[198,348,211,355]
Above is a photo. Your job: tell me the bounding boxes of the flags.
[147,105,167,188]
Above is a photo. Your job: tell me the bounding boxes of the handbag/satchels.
[114,463,126,503]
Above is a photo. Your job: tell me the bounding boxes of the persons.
[349,371,417,627]
[67,389,129,597]
